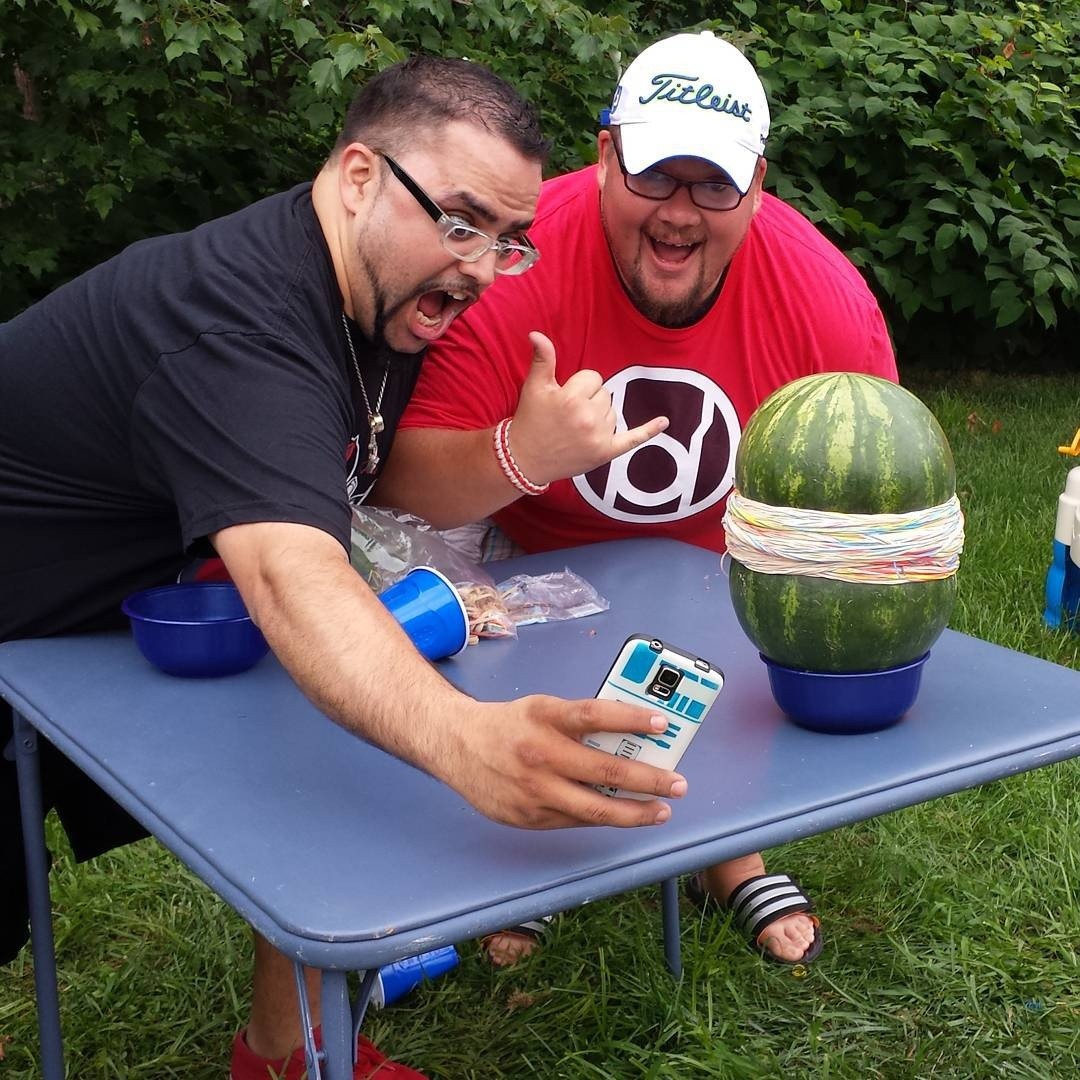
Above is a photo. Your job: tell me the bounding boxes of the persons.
[370,29,901,973]
[0,69,688,1080]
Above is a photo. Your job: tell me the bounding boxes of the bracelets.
[493,416,549,495]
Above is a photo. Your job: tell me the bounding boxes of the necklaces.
[338,314,393,473]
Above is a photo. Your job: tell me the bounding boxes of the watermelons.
[726,370,960,677]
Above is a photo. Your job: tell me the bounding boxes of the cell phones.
[579,634,725,801]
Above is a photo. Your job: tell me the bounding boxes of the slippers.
[726,874,823,966]
[480,912,554,970]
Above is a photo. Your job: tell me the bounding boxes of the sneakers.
[230,1021,424,1079]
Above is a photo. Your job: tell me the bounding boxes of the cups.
[358,945,459,1010]
[376,567,470,664]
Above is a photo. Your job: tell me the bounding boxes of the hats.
[609,32,771,194]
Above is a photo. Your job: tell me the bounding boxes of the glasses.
[610,130,746,210]
[377,152,538,277]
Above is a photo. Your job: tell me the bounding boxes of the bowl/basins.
[757,651,932,734]
[120,582,270,680]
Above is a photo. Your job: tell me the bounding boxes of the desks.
[0,536,1080,1079]
